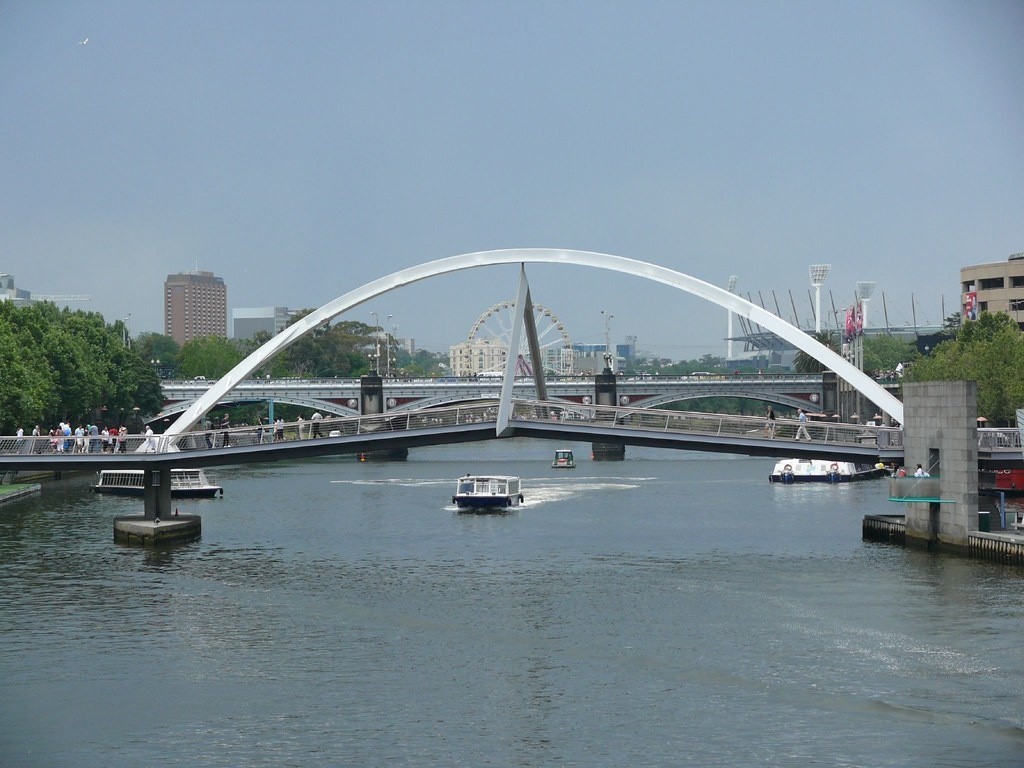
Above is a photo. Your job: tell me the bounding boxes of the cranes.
[29,293,95,302]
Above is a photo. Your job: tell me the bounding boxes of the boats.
[88,469,222,498]
[768,457,893,484]
[550,449,576,468]
[451,473,524,509]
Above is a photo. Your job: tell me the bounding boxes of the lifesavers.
[830,463,839,471]
[784,464,792,472]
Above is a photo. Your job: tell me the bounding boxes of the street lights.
[123,318,128,347]
[726,275,739,359]
[370,312,381,375]
[389,357,396,373]
[367,354,377,369]
[127,338,131,349]
[839,307,848,356]
[857,280,878,327]
[809,264,831,342]
[600,309,608,368]
[132,407,140,434]
[386,315,393,383]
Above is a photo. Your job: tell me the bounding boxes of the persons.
[763,405,776,439]
[205,418,213,448]
[914,464,922,477]
[50,420,127,454]
[312,410,324,438]
[894,463,906,477]
[298,414,305,440]
[795,409,811,442]
[33,426,40,436]
[221,413,231,447]
[274,416,286,442]
[16,426,24,453]
[257,418,264,443]
[145,425,153,435]
